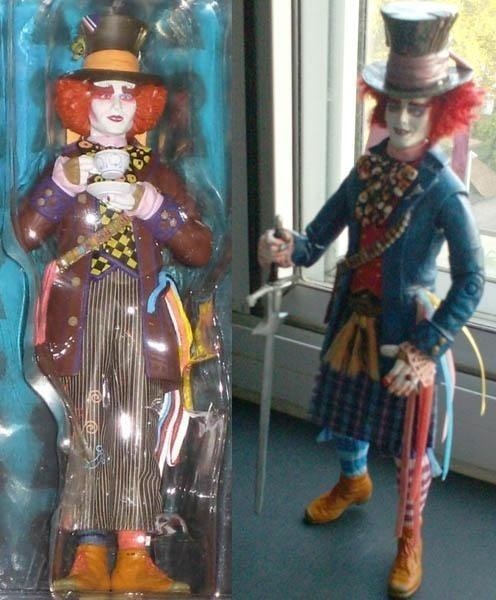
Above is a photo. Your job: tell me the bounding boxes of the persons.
[252,7,487,599]
[17,11,220,600]
